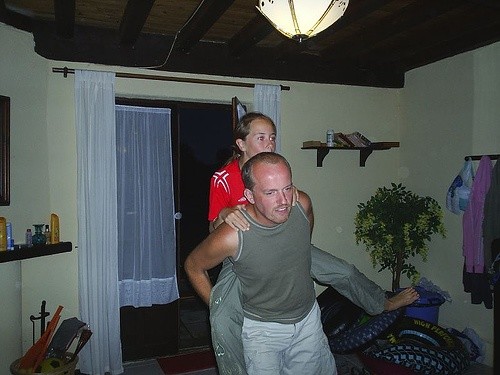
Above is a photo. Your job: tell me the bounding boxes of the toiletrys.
[2,212,63,254]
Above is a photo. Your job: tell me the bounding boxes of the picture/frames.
[1,95,10,206]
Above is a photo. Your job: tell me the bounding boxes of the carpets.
[158,350,218,375]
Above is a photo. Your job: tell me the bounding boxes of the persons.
[208,112,419,375]
[184,152,337,375]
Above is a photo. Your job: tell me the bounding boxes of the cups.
[327,130,334,146]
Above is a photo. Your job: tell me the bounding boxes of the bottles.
[25,213,60,248]
[0,216,14,251]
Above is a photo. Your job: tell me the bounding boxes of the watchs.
[212,217,218,229]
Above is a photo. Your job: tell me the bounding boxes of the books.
[334,131,372,147]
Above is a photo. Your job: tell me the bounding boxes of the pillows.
[361,339,471,375]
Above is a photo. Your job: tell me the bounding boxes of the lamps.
[255,0,350,42]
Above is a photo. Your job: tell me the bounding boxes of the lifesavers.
[319,289,403,354]
[357,317,471,375]
